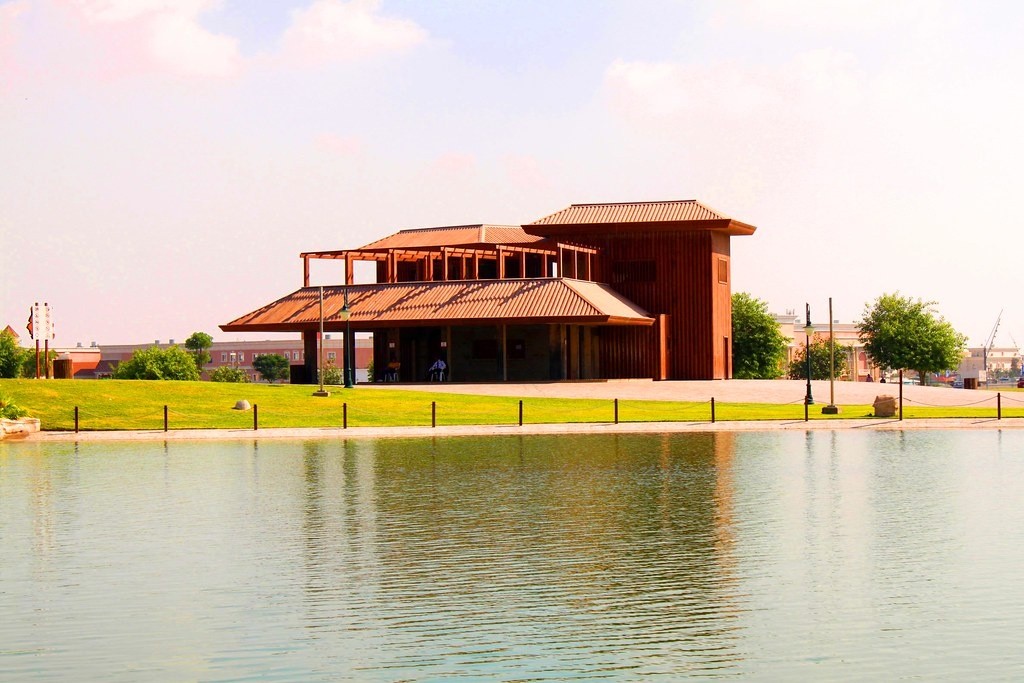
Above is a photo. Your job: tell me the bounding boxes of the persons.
[880,375,886,383]
[429,355,446,383]
[386,359,400,380]
[866,374,873,382]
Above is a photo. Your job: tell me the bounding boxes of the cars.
[1017,378,1024,388]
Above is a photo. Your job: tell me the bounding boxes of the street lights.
[802,302,816,405]
[339,286,354,389]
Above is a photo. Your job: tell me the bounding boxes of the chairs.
[431,372,446,382]
[385,363,401,382]
